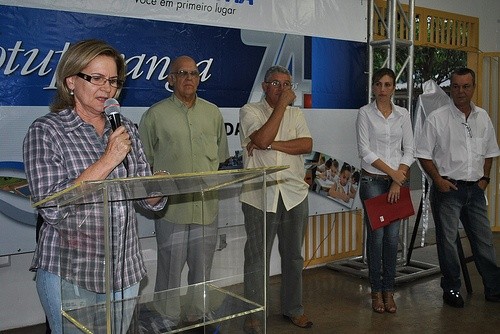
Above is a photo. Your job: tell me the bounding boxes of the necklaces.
[377,103,393,116]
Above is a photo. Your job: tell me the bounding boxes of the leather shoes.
[484,290,500,302]
[442,289,465,308]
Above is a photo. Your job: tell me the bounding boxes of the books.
[364,185,415,230]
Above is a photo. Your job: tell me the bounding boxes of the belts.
[448,179,478,185]
[361,169,391,180]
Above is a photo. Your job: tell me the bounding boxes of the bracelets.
[153,169,170,175]
[480,176,490,184]
[267,144,272,150]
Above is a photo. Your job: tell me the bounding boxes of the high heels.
[371,291,384,313]
[381,291,397,312]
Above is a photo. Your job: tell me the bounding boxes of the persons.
[22,41,172,334]
[239,66,313,334]
[139,56,230,334]
[413,67,499,308]
[312,157,360,203]
[355,68,417,313]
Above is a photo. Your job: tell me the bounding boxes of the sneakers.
[183,319,219,334]
[283,313,314,328]
[243,318,264,334]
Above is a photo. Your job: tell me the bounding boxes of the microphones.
[104,98,128,170]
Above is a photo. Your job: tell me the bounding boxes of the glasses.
[266,80,292,87]
[170,70,199,78]
[76,73,125,88]
[462,123,473,138]
